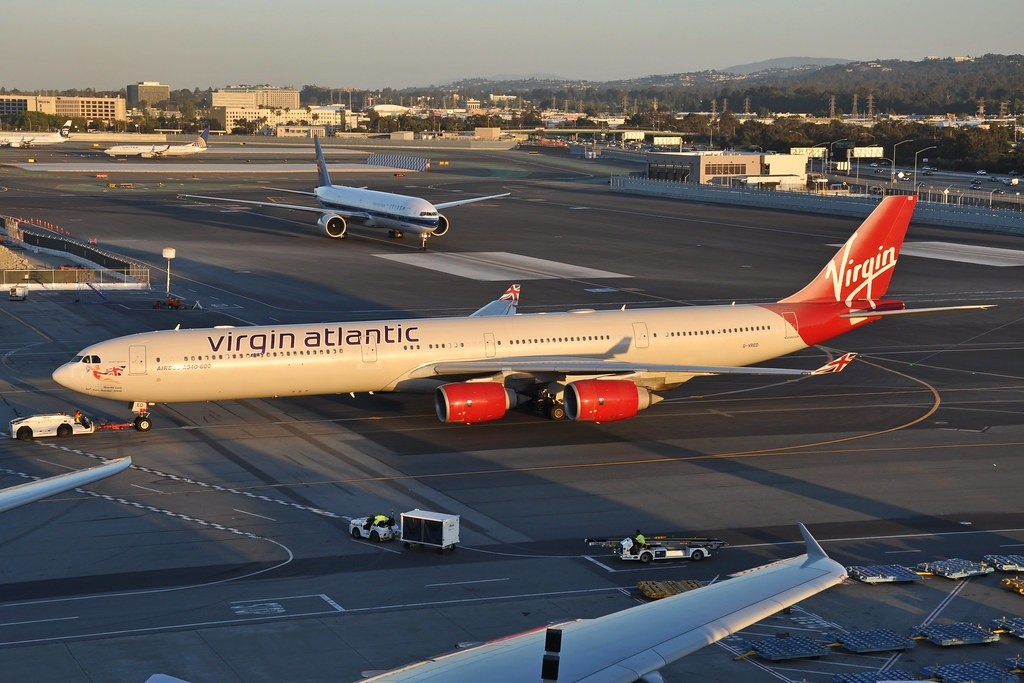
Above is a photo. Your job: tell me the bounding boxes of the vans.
[977,170,986,175]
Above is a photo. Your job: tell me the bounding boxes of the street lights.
[989,188,1000,208]
[788,129,937,192]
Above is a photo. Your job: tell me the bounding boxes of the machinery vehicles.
[618,538,711,563]
[9,412,94,441]
[9,285,29,301]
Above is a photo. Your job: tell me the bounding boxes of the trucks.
[349,515,400,543]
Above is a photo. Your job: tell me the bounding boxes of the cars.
[553,135,694,153]
[987,176,998,182]
[1002,178,1015,186]
[971,179,982,184]
[993,187,1005,194]
[869,162,938,187]
[969,184,981,190]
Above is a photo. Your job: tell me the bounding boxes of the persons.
[74,410,90,429]
[630,528,646,555]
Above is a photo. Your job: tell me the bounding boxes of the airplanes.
[176,134,511,251]
[52,195,998,425]
[143,522,849,683]
[103,128,210,158]
[0,119,73,148]
